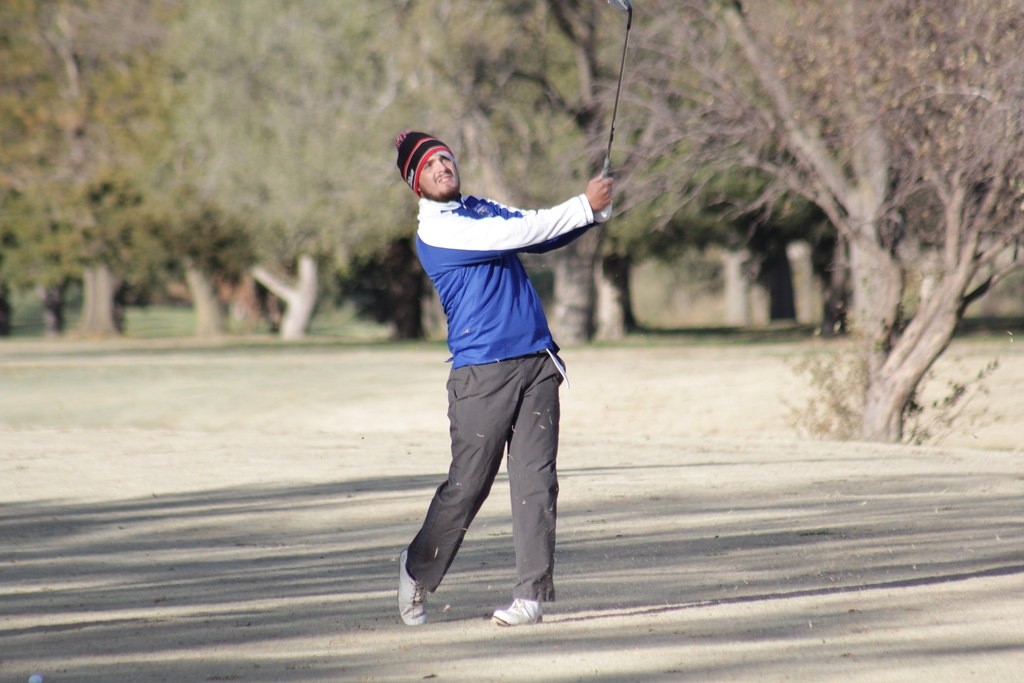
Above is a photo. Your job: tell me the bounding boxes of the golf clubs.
[602,0,632,179]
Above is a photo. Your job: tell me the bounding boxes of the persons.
[395,131,613,626]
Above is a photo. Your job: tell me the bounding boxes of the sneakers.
[490,598,543,627]
[397,548,429,626]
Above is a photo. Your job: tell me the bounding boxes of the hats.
[395,129,456,199]
